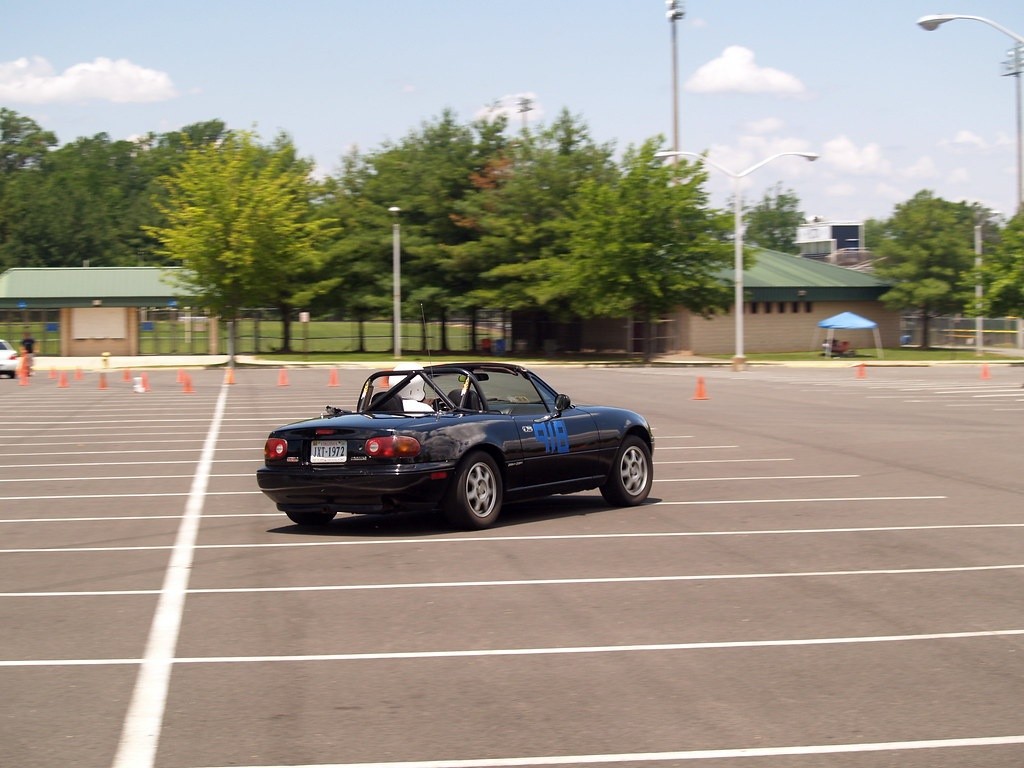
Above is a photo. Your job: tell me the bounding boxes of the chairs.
[448,389,481,414]
[371,392,404,415]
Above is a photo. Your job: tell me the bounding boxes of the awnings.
[817,312,884,358]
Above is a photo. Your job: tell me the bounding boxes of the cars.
[0,338,23,377]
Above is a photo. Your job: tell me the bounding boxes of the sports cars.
[256,360,656,529]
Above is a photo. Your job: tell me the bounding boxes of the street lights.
[387,205,407,362]
[916,13,1024,226]
[653,150,823,370]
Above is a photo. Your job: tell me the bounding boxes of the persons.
[21,332,37,376]
[389,363,435,416]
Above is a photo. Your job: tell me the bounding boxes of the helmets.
[389,363,427,402]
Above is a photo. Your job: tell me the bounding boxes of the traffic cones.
[224,368,239,384]
[140,372,152,392]
[74,366,85,381]
[327,367,340,387]
[183,374,196,392]
[46,364,58,378]
[18,367,31,386]
[380,368,391,388]
[57,369,71,389]
[121,367,134,381]
[857,364,866,381]
[177,367,188,384]
[277,368,291,387]
[98,371,110,389]
[693,376,712,400]
[980,361,991,379]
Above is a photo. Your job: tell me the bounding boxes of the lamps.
[92,299,102,306]
[798,290,807,296]
